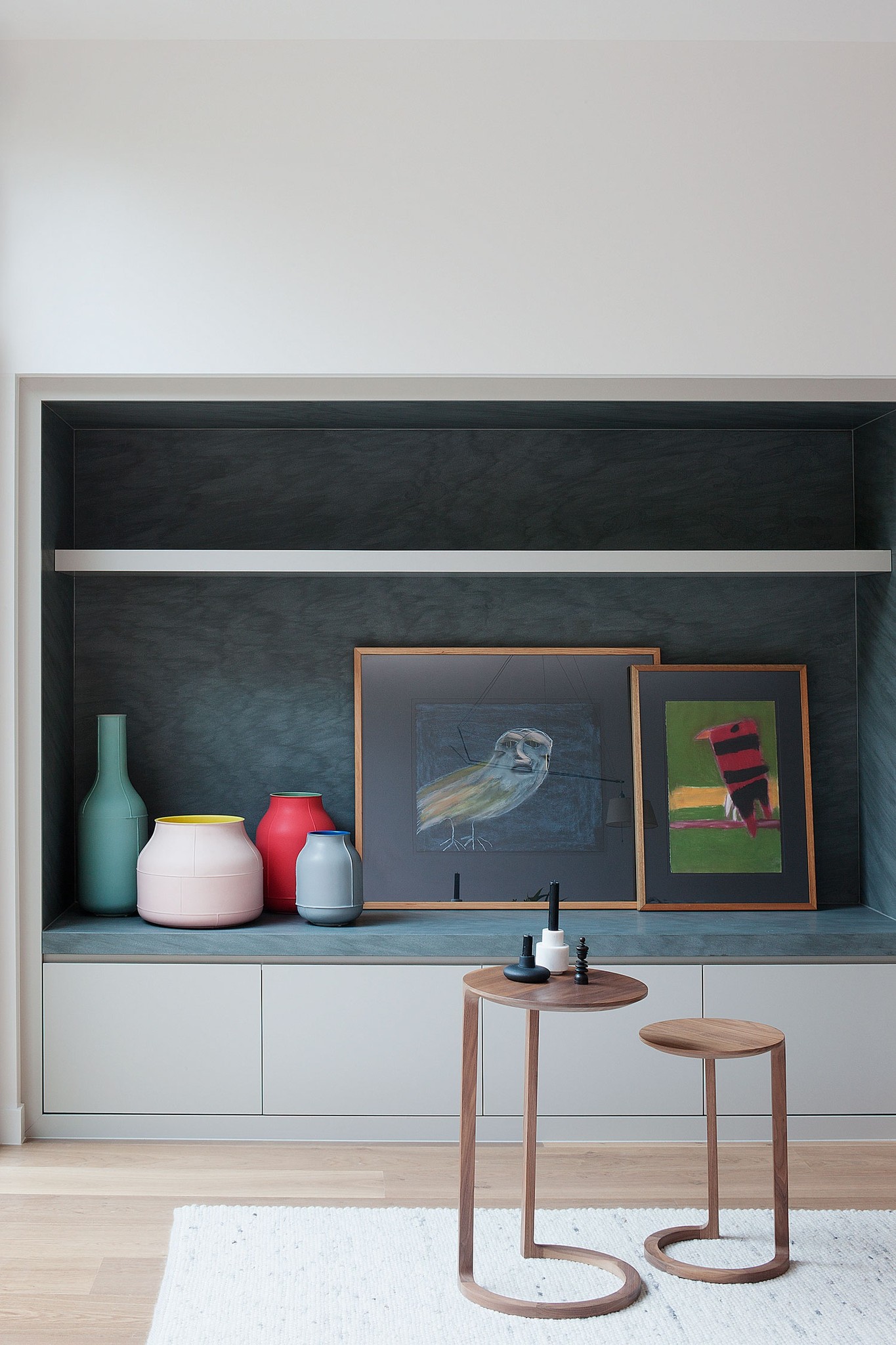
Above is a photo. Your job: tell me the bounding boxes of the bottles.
[136,815,264,931]
[255,791,336,915]
[74,713,148,917]
[295,830,365,927]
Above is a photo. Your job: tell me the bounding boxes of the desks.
[457,966,649,1319]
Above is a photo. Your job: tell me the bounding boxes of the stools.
[638,1018,790,1284]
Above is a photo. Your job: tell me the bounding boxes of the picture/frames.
[630,664,818,910]
[354,648,660,910]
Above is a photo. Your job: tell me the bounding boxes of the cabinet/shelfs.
[37,400,896,1139]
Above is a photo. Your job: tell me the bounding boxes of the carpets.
[145,1203,896,1345]
